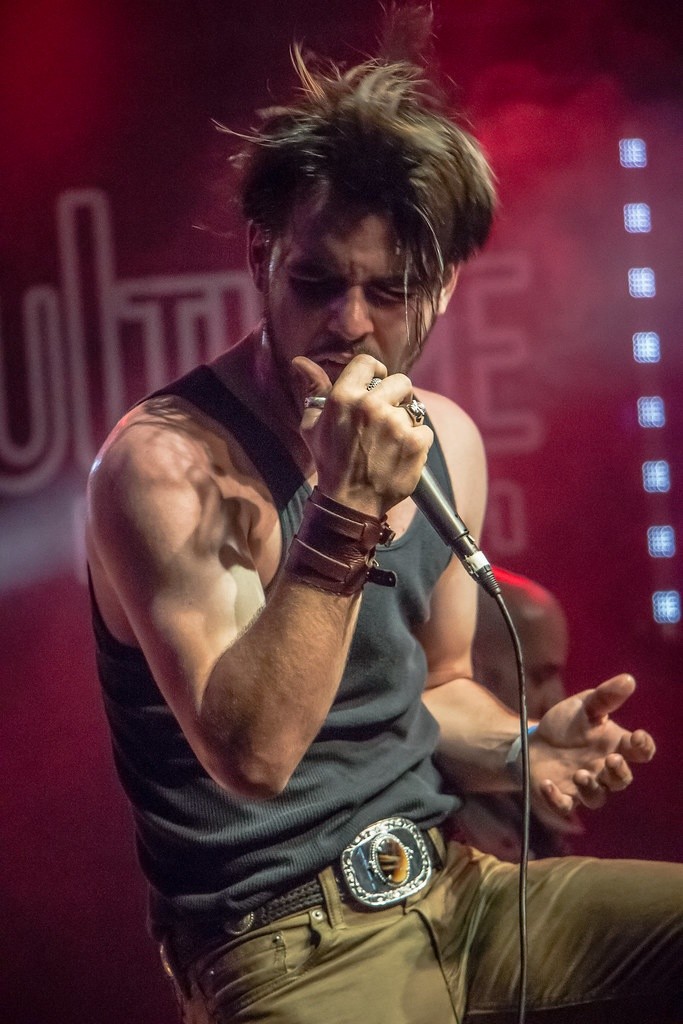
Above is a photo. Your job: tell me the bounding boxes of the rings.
[400,398,426,426]
[303,396,327,409]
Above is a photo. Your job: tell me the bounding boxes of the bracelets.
[506,724,538,780]
[282,482,398,598]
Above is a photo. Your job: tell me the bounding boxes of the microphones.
[369,377,503,600]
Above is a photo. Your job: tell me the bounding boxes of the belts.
[173,817,443,971]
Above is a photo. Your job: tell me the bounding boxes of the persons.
[85,29,682,1023]
[434,567,601,864]
